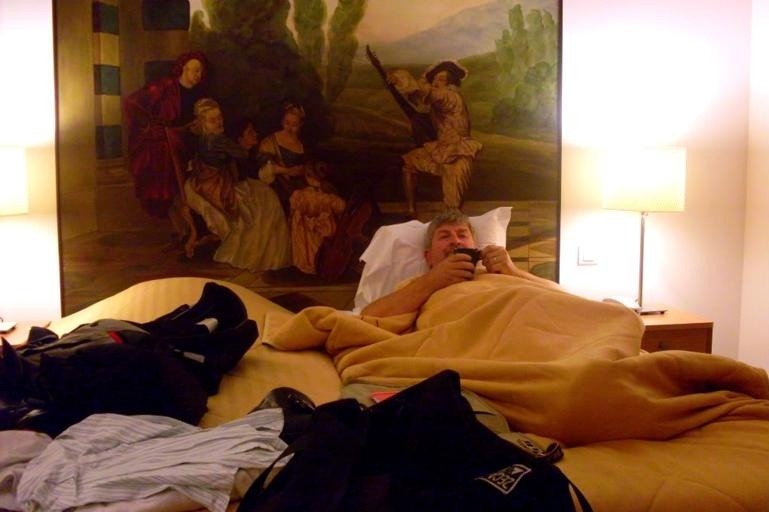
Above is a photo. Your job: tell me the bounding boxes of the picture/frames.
[50,0,561,317]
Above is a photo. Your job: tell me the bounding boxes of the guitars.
[365,44,438,147]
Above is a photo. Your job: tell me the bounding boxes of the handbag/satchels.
[236,369,595,511]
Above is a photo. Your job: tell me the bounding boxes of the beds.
[47,266,767,509]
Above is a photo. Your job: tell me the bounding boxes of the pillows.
[353,207,513,310]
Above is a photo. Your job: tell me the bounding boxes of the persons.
[385,58,482,217]
[359,210,559,320]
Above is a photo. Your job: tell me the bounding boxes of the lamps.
[599,137,689,314]
[0,143,29,333]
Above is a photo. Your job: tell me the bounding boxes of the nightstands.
[632,301,713,354]
[0,321,49,348]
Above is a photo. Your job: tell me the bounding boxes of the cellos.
[315,167,386,282]
